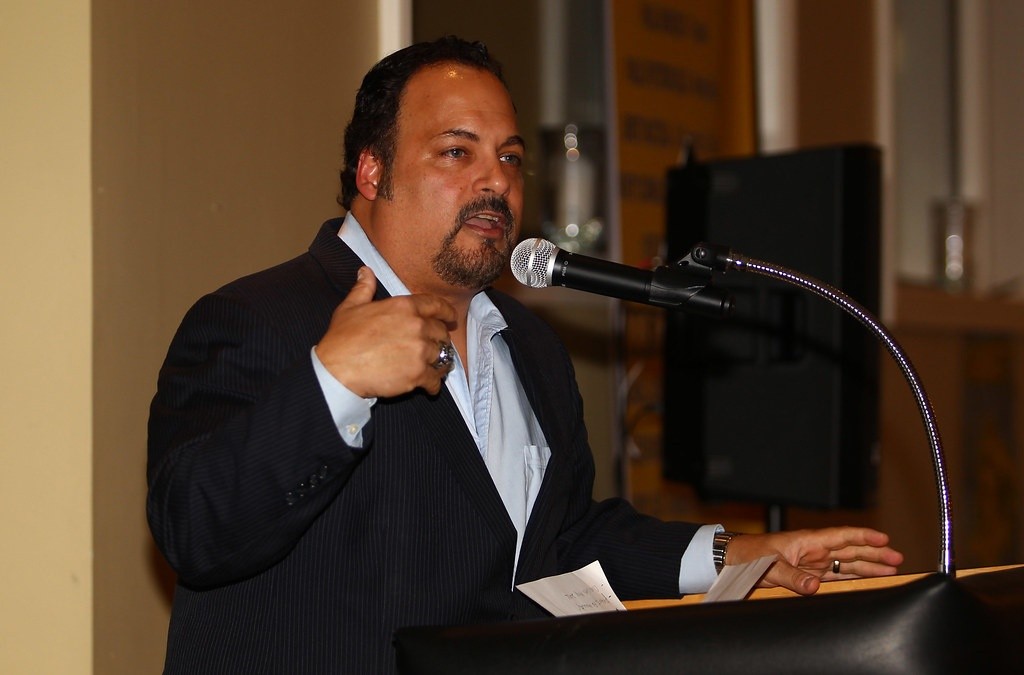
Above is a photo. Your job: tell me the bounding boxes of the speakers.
[656,139,885,511]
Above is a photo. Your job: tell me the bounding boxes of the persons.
[147,40,905,675]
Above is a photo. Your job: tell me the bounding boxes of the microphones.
[509,238,733,317]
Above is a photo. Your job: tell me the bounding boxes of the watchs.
[713,530,743,574]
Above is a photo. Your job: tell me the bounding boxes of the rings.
[431,341,456,370]
[833,560,840,574]
